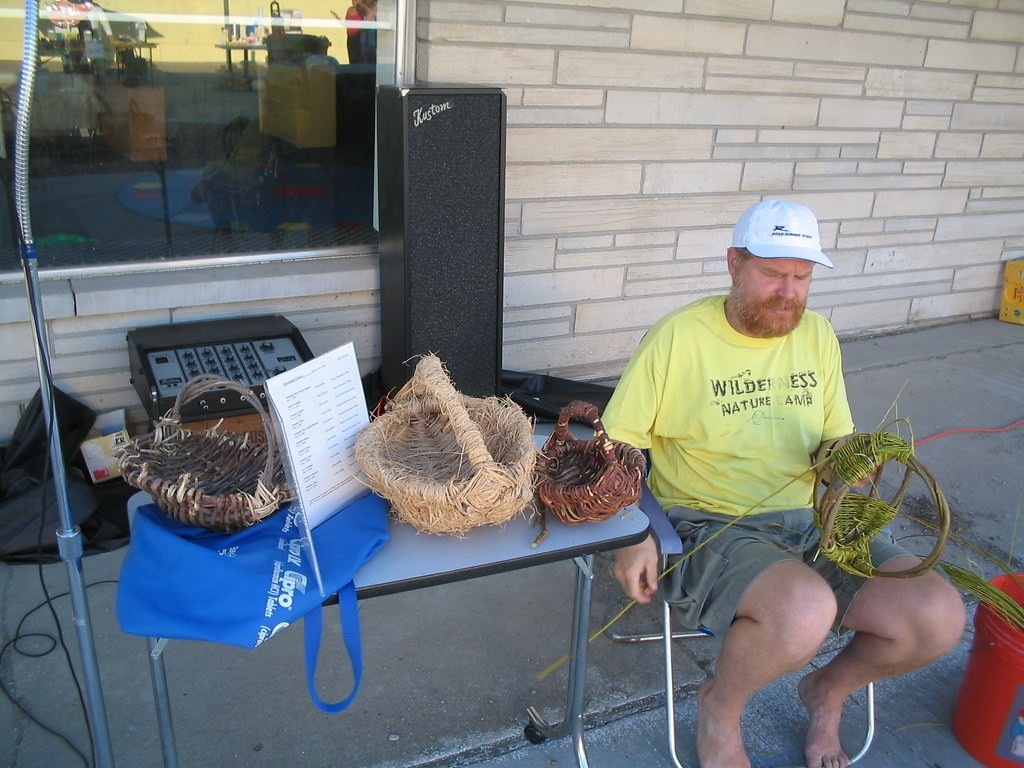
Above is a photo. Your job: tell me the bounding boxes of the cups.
[49,33,55,42]
[56,33,62,41]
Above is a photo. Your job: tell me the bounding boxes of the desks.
[127,434,651,768]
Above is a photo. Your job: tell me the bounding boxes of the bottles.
[84,33,92,41]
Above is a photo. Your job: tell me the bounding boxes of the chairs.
[601,475,876,768]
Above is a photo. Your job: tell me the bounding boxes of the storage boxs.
[98,77,169,164]
[256,58,339,149]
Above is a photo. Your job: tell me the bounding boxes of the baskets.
[533,399,645,526]
[119,374,289,529]
[355,355,532,534]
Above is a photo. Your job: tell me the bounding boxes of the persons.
[331,0,377,65]
[593,199,967,768]
[191,54,345,253]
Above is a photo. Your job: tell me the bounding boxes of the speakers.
[375,82,506,396]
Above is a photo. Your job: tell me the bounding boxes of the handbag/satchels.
[115,493,390,714]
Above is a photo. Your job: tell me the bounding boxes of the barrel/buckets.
[953,573,1024,768]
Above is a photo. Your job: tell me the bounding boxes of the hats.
[732,198,835,271]
